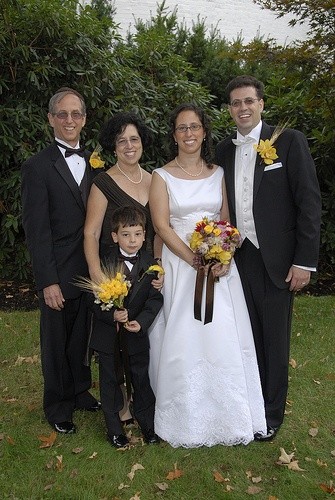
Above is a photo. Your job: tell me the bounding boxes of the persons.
[84,113,163,450]
[21,90,105,434]
[149,104,267,450]
[214,75,321,441]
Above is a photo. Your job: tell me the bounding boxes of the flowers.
[75,263,134,310]
[90,149,105,169]
[137,265,165,283]
[254,133,279,164]
[188,218,240,283]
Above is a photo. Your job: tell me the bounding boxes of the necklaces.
[116,161,143,184]
[175,156,203,177]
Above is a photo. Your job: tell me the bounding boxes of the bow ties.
[65,147,86,158]
[117,252,138,265]
[232,135,258,145]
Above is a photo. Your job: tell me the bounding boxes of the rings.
[302,282,305,285]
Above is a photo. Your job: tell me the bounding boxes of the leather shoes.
[254,425,281,442]
[142,429,161,446]
[110,434,130,449]
[54,421,76,434]
[85,399,104,412]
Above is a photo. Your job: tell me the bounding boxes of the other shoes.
[121,418,135,426]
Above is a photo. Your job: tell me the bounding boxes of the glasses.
[49,112,84,120]
[118,137,141,147]
[232,98,259,107]
[176,123,202,133]
